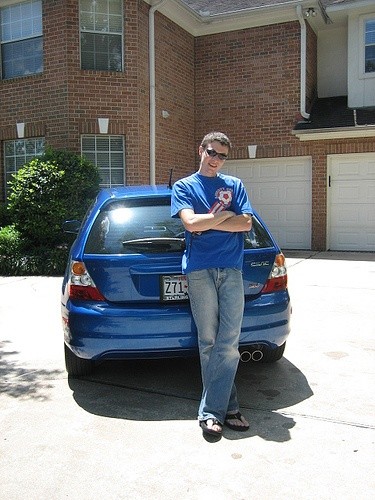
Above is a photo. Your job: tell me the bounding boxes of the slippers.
[199,420,223,436]
[224,412,249,431]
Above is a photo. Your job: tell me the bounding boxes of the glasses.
[202,147,228,160]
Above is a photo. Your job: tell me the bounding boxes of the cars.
[61,183,293,379]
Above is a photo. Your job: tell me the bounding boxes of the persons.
[171,132,253,436]
